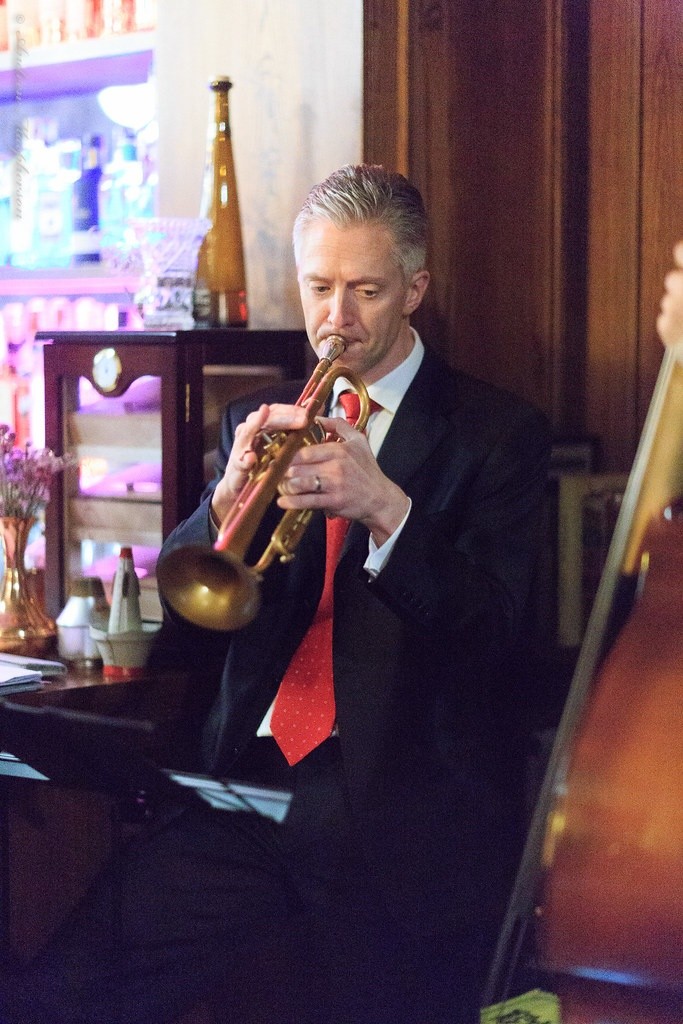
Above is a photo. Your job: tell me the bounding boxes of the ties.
[270,393,383,767]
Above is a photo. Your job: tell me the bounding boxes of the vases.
[0,517,59,660]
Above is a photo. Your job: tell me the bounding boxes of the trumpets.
[154,333,372,634]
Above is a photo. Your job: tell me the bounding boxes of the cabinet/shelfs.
[34,327,315,620]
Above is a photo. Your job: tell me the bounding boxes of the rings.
[316,477,321,494]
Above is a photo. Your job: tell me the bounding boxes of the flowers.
[0,424,62,516]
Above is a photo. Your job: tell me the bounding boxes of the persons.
[0,166,554,1023]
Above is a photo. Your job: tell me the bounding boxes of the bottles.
[191,79,250,329]
[2,116,148,267]
[108,547,143,634]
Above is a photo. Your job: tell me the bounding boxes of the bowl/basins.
[90,623,162,676]
